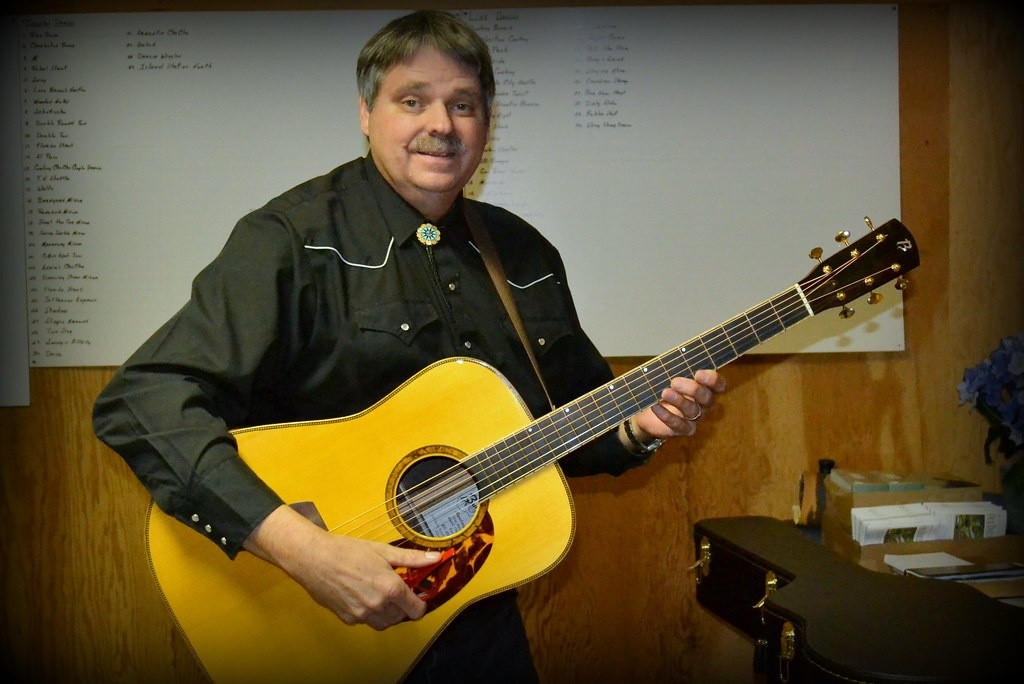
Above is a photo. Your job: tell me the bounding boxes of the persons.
[91,8,729,684]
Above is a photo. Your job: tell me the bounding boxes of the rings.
[684,403,703,422]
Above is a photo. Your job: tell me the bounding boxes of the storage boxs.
[821,475,1024,608]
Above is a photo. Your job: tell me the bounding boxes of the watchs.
[622,419,666,456]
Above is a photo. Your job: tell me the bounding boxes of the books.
[903,561,1024,581]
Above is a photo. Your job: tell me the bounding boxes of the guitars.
[141,212,924,684]
